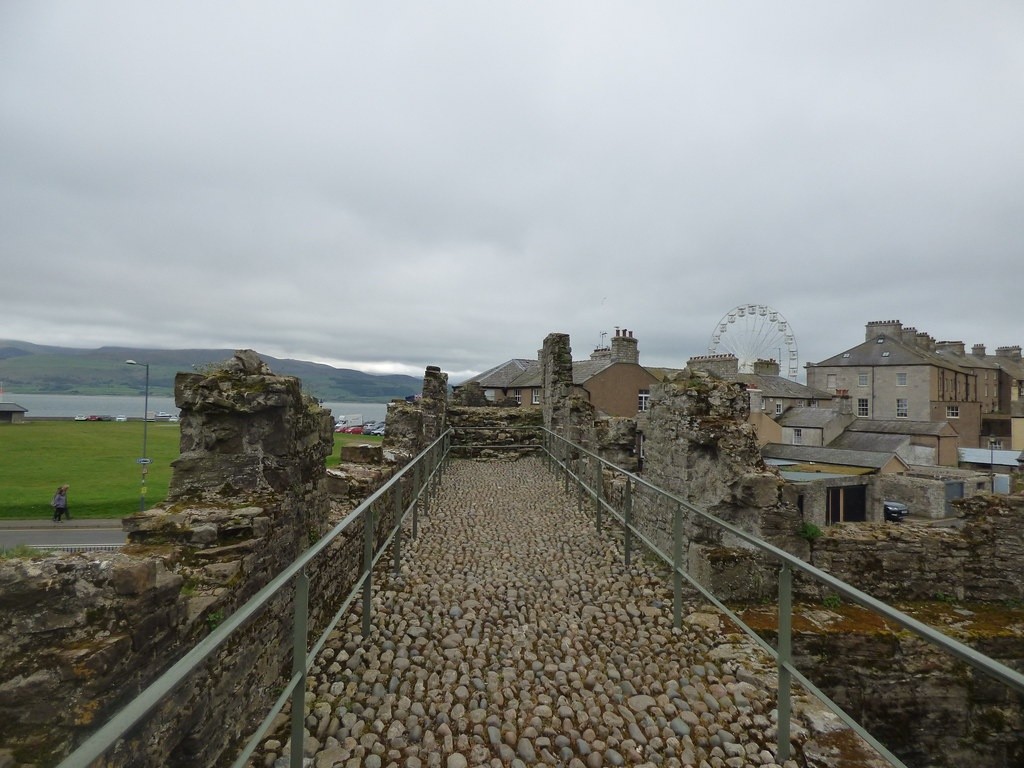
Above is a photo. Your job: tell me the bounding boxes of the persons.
[51,484,73,523]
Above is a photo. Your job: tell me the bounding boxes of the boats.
[153,412,173,422]
[141,411,180,422]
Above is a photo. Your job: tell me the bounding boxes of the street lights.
[125,359,149,511]
[990,434,996,494]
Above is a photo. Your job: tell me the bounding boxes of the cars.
[884,501,912,522]
[334,413,386,436]
[88,415,99,421]
[116,415,128,422]
[100,415,112,421]
[75,414,87,421]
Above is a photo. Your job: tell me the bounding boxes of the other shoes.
[57,520,63,523]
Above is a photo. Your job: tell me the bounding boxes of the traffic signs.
[136,457,152,464]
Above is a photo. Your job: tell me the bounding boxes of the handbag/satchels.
[51,499,56,506]
